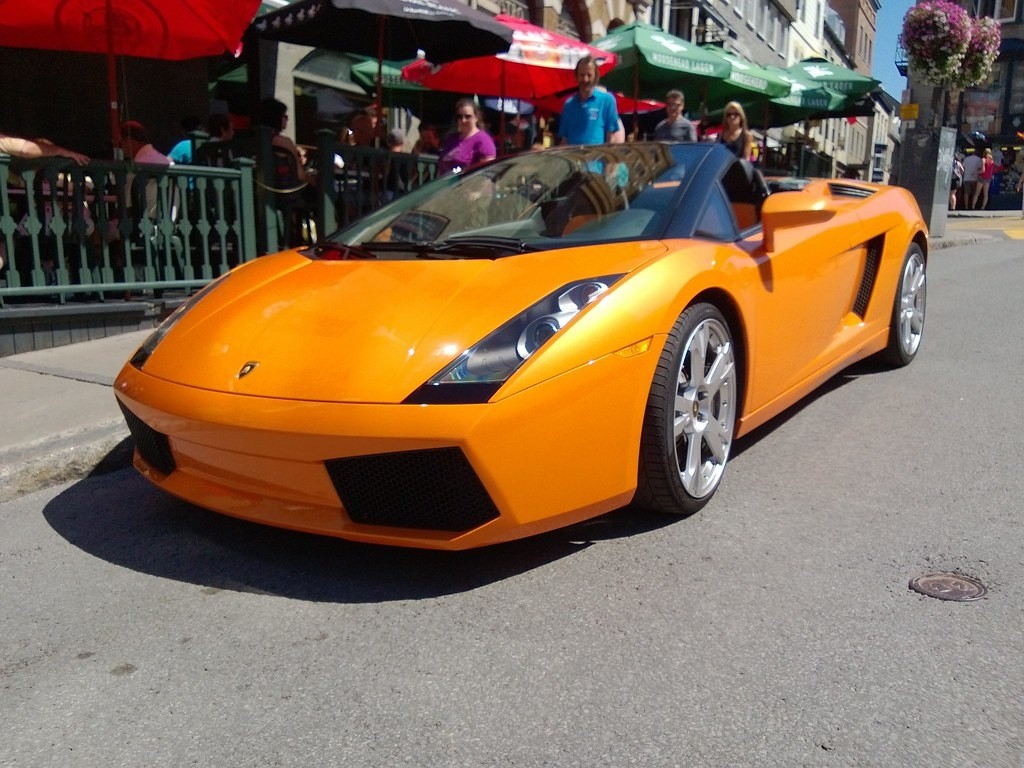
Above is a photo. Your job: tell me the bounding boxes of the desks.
[7,188,118,236]
[306,166,384,182]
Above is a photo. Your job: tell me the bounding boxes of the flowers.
[957,12,1002,90]
[902,0,972,89]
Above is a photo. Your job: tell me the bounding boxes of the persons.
[0,133,91,166]
[120,120,175,164]
[412,121,439,152]
[437,99,496,177]
[166,111,234,165]
[350,115,401,151]
[273,100,325,245]
[950,145,1024,210]
[715,102,753,160]
[654,89,697,141]
[558,56,619,145]
[956,122,986,151]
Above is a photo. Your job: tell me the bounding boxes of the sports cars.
[117,142,929,547]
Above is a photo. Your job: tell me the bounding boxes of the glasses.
[727,113,740,117]
[456,114,471,119]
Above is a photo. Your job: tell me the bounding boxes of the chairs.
[548,172,614,219]
[685,155,769,231]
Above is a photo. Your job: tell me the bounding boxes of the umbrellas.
[0,0,883,158]
